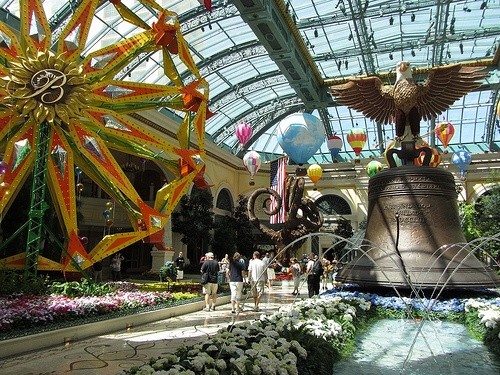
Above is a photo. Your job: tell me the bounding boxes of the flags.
[269,156,286,225]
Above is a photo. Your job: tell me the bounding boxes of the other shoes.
[323,287,328,290]
[203,308,215,312]
[292,292,300,295]
[232,310,242,313]
[253,307,262,312]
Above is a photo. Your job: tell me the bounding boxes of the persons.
[322,264,329,290]
[275,255,297,274]
[175,252,185,282]
[302,254,308,274]
[111,252,124,281]
[200,252,220,311]
[306,254,323,298]
[93,263,103,282]
[221,253,230,284]
[290,259,301,295]
[332,263,343,290]
[248,251,267,312]
[322,255,337,279]
[227,251,244,313]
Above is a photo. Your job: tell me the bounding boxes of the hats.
[205,252,214,257]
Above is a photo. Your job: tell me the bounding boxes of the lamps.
[285,0,487,70]
[201,24,212,32]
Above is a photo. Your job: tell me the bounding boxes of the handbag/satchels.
[110,263,115,270]
[202,261,210,284]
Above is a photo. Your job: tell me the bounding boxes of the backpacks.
[310,259,323,277]
